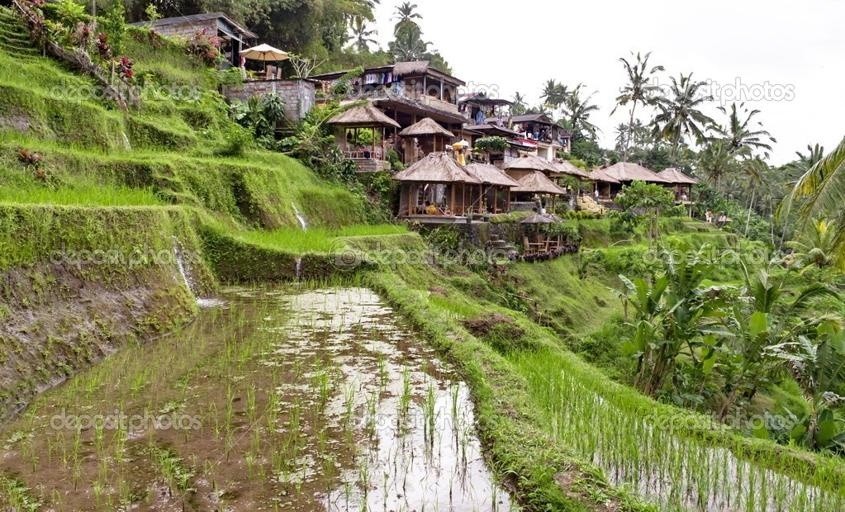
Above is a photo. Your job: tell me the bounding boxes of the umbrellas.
[238,43,289,74]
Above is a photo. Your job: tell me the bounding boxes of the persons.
[718,213,726,228]
[517,124,551,143]
[346,129,371,159]
